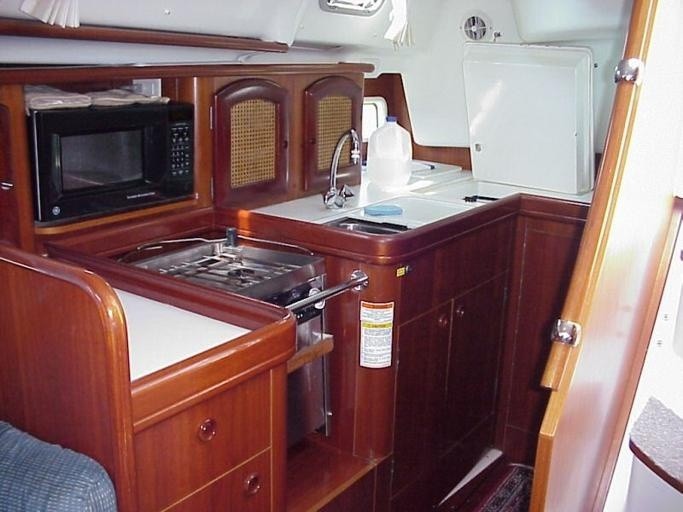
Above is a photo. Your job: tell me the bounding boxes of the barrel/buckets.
[366,116,414,197]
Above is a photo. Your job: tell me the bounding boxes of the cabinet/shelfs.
[3,255,292,510]
[239,190,520,510]
[494,193,595,466]
[0,64,210,250]
[200,60,373,210]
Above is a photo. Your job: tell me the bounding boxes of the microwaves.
[26,97,197,231]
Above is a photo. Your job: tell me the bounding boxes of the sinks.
[322,216,410,236]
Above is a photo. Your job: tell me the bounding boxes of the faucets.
[323,128,361,211]
